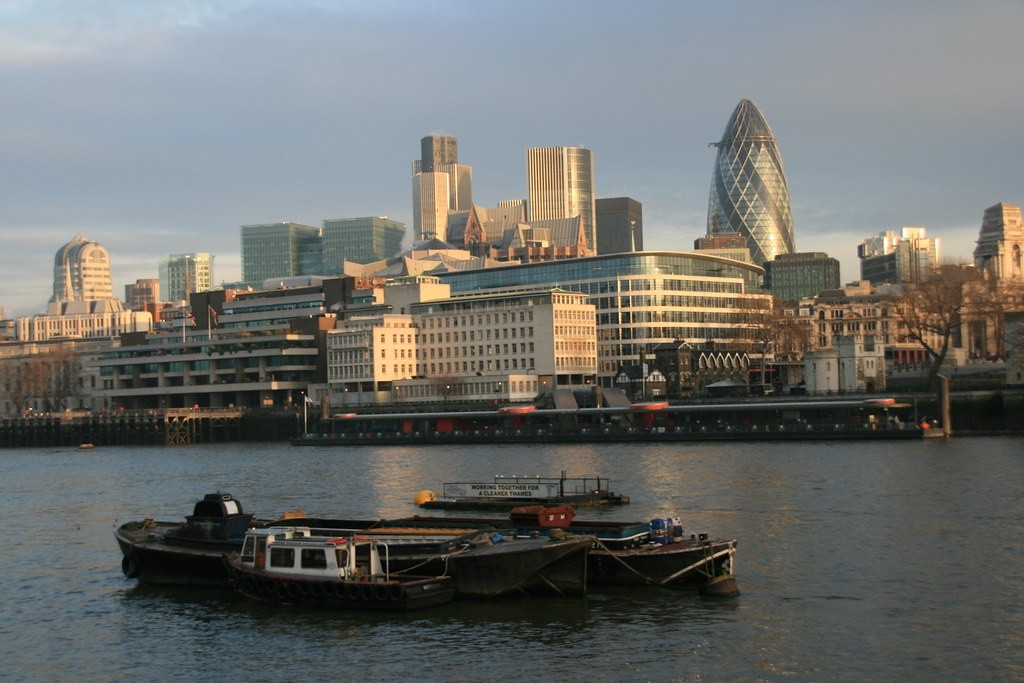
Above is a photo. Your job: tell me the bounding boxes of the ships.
[291,395,945,447]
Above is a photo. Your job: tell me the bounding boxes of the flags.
[185,305,218,327]
[306,397,312,403]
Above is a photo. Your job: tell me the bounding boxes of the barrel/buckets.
[673,526,682,537]
[649,518,674,546]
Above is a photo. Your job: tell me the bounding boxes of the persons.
[228,402,234,413]
[23,400,124,415]
[194,402,200,413]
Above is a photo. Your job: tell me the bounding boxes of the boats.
[419,469,630,512]
[114,489,739,614]
